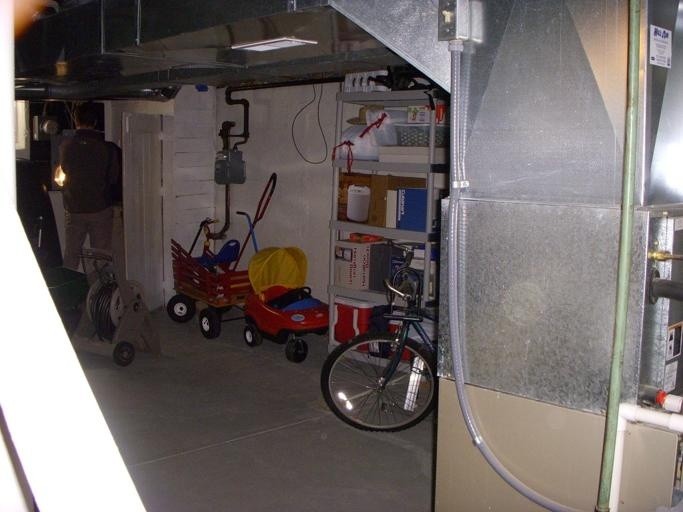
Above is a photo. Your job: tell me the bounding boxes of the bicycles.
[319,240,436,432]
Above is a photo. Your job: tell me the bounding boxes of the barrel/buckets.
[347,185,370,222]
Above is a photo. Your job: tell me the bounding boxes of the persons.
[57,102,123,277]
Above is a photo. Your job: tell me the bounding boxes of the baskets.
[393,122,450,146]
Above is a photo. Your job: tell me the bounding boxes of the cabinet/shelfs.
[327,90,449,382]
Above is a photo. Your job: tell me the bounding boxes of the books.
[377,144,448,164]
[384,186,426,230]
[408,258,436,271]
[413,247,435,260]
